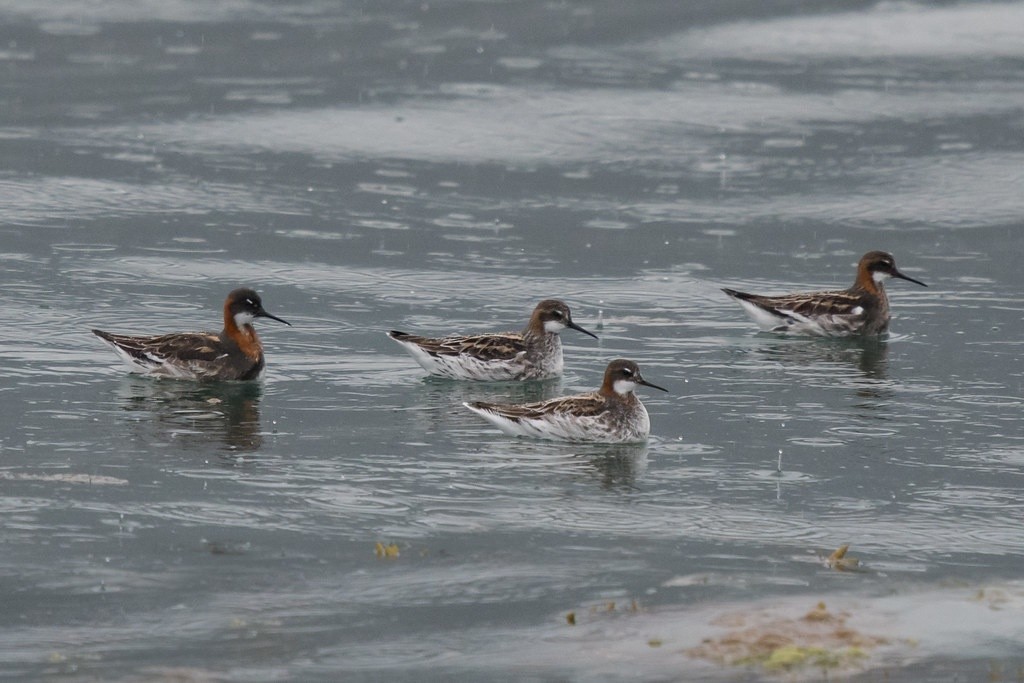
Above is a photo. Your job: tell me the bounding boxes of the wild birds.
[465,360,668,445]
[720,250,927,338]
[90,288,293,382]
[386,300,598,382]
